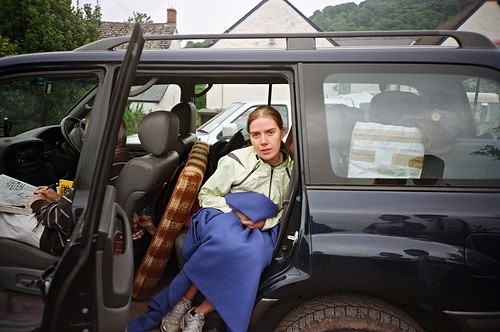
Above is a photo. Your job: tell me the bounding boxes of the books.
[0,174,40,215]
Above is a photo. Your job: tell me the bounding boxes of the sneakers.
[162,297,191,332]
[182,306,205,332]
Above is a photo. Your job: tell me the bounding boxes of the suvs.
[0,28,500,332]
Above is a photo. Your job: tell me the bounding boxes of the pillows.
[346,120,424,180]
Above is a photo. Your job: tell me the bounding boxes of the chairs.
[115,101,199,231]
[324,89,425,180]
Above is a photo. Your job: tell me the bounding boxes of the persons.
[160,106,295,332]
[0,117,133,255]
